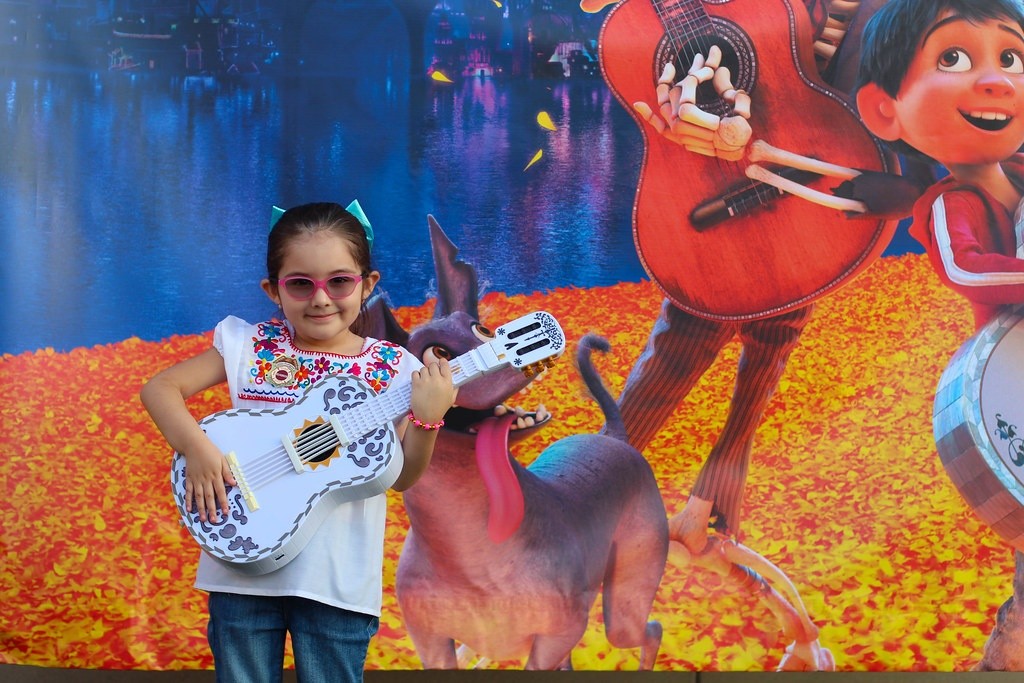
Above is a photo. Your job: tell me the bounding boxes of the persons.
[139,198,459,683]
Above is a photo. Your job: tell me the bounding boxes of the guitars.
[168,308,567,578]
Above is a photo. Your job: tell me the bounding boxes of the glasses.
[269,272,367,301]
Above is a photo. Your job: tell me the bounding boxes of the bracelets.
[408,412,445,431]
